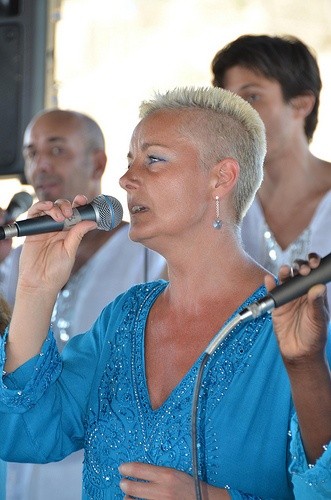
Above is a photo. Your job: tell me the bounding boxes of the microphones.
[0,195,123,239]
[7,192,33,221]
[237,251,331,320]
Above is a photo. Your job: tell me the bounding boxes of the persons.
[209,33,331,320]
[264,250,330,500]
[0,84,296,499]
[0,108,168,499]
